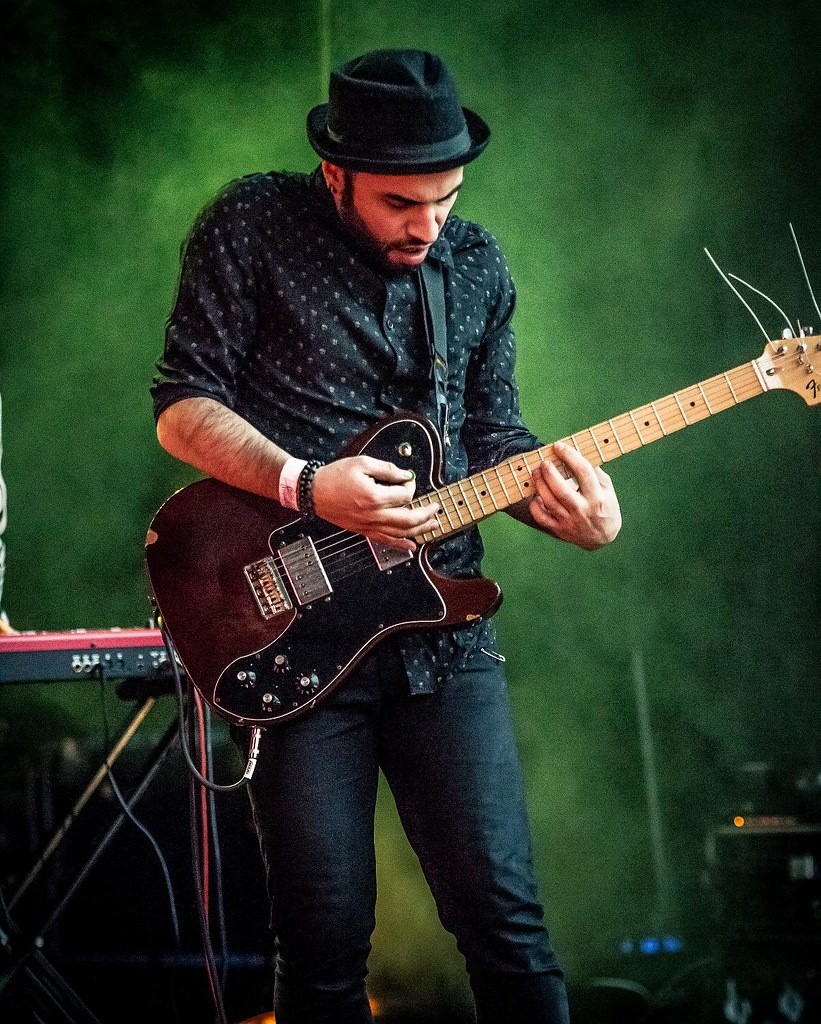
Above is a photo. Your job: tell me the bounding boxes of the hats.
[305,48,491,176]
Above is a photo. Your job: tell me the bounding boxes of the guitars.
[143,326,818,727]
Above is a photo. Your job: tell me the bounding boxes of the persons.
[147,51,624,1024]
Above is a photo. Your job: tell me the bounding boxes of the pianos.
[0,626,183,689]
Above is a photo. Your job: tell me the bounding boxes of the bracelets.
[278,456,308,512]
[298,459,325,523]
